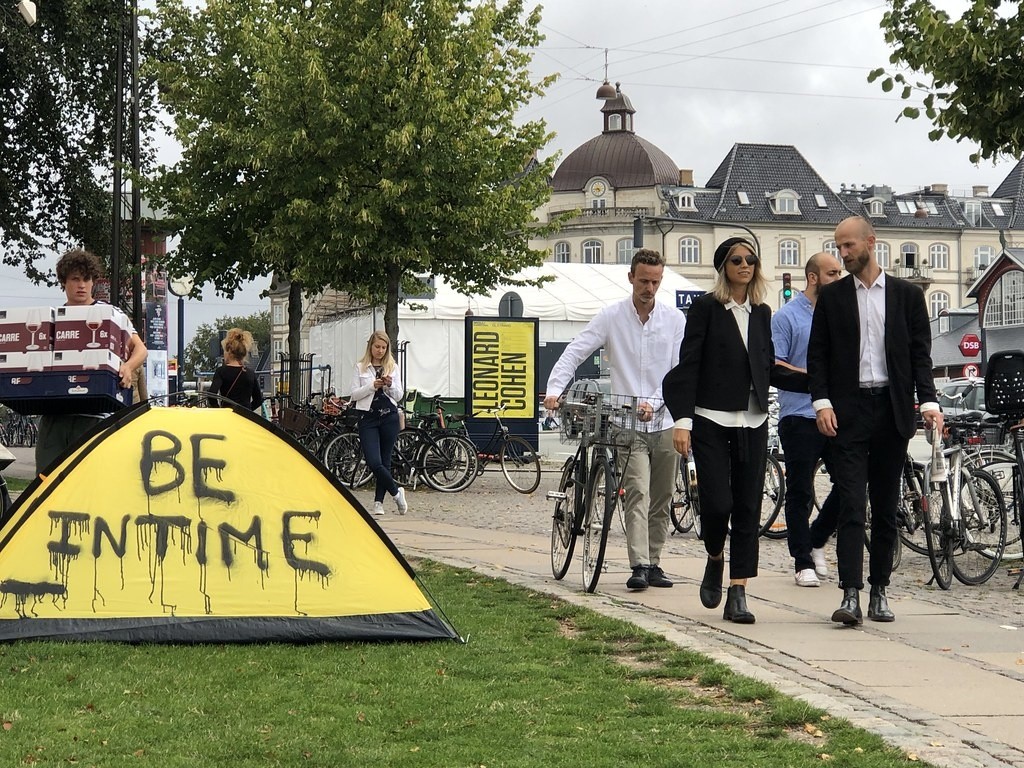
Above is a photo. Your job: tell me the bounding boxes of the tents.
[285,261,709,419]
[0,388,468,644]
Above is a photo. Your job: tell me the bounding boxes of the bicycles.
[921,392,1006,589]
[263,390,541,494]
[560,455,627,536]
[546,389,666,593]
[961,351,1024,590]
[0,404,39,448]
[670,445,815,540]
[812,452,946,571]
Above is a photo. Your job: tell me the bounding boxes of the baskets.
[559,389,665,453]
[282,407,310,433]
[950,422,1005,447]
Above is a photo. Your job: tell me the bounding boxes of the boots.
[831,587,863,624]
[868,585,895,622]
[700,551,724,609]
[723,584,755,624]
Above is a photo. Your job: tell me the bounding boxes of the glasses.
[731,255,757,266]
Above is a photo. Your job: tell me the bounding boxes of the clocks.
[170,269,194,296]
[591,180,605,197]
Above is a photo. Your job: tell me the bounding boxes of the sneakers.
[626,563,649,588]
[373,501,384,515]
[812,548,827,580]
[393,487,407,515]
[795,569,820,587]
[649,564,673,587]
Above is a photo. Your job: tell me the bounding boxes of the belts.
[860,386,889,397]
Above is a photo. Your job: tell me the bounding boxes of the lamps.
[465,296,473,315]
[596,48,617,100]
[915,196,928,218]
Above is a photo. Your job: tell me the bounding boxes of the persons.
[807,216,945,625]
[35,251,148,479]
[350,331,408,515]
[544,249,687,588]
[771,253,842,588]
[207,328,264,411]
[666,237,811,623]
[132,365,147,404]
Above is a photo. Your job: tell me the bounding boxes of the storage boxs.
[0,306,132,417]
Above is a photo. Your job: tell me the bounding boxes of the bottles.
[398,408,405,430]
[685,449,699,500]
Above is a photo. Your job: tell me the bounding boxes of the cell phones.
[381,377,386,381]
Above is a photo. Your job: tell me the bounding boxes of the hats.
[713,237,756,273]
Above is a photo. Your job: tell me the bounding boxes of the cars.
[913,390,925,428]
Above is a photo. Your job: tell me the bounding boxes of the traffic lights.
[783,273,791,299]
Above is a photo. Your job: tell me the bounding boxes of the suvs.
[925,377,1024,446]
[561,374,611,439]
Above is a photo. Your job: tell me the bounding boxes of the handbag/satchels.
[398,407,405,432]
